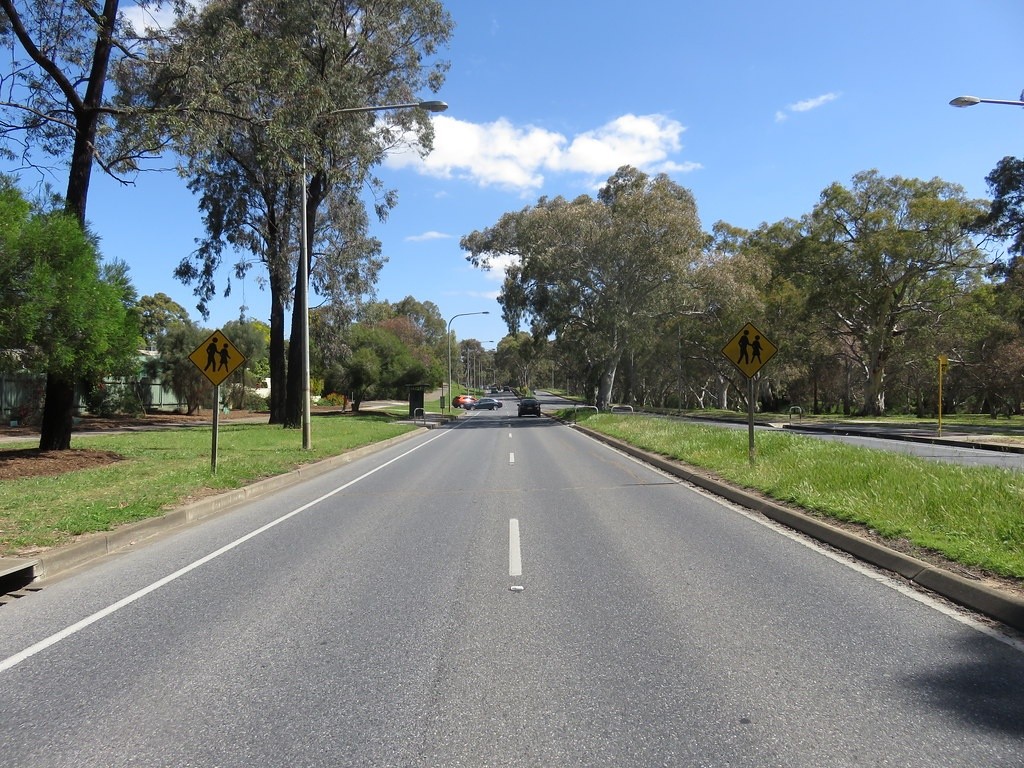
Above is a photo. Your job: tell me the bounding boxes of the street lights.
[467,340,494,395]
[300,100,449,451]
[447,312,490,415]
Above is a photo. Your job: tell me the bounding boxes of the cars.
[517,398,541,417]
[466,397,502,411]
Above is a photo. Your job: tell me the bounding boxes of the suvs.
[503,386,510,392]
[491,387,499,394]
[452,394,476,408]
